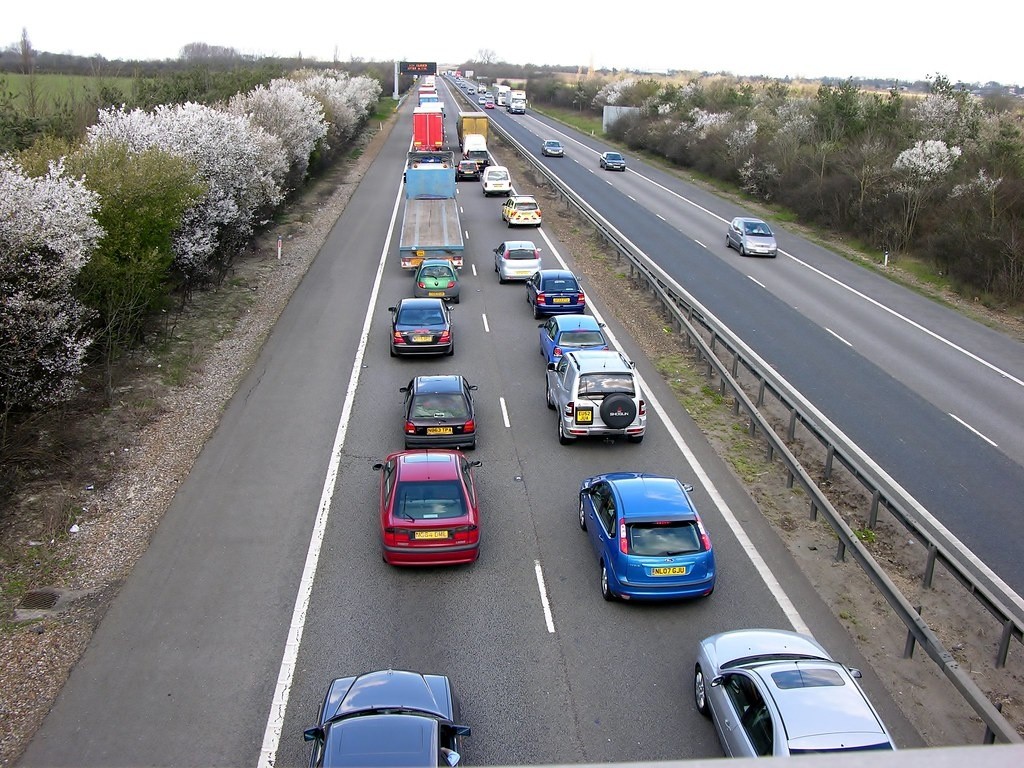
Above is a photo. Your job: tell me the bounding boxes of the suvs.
[546,350,647,446]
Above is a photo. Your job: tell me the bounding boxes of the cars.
[387,296,455,360]
[576,472,717,609]
[461,147,488,170]
[526,270,585,320]
[600,151,626,172]
[499,193,542,230]
[539,139,566,158]
[538,315,609,363]
[371,449,484,569]
[726,216,777,259]
[453,159,483,183]
[411,257,460,306]
[693,629,898,756]
[491,239,543,284]
[305,665,471,767]
[442,69,511,110]
[479,165,511,197]
[399,374,478,448]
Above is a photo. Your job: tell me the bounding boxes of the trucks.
[398,74,490,286]
[503,90,529,115]
